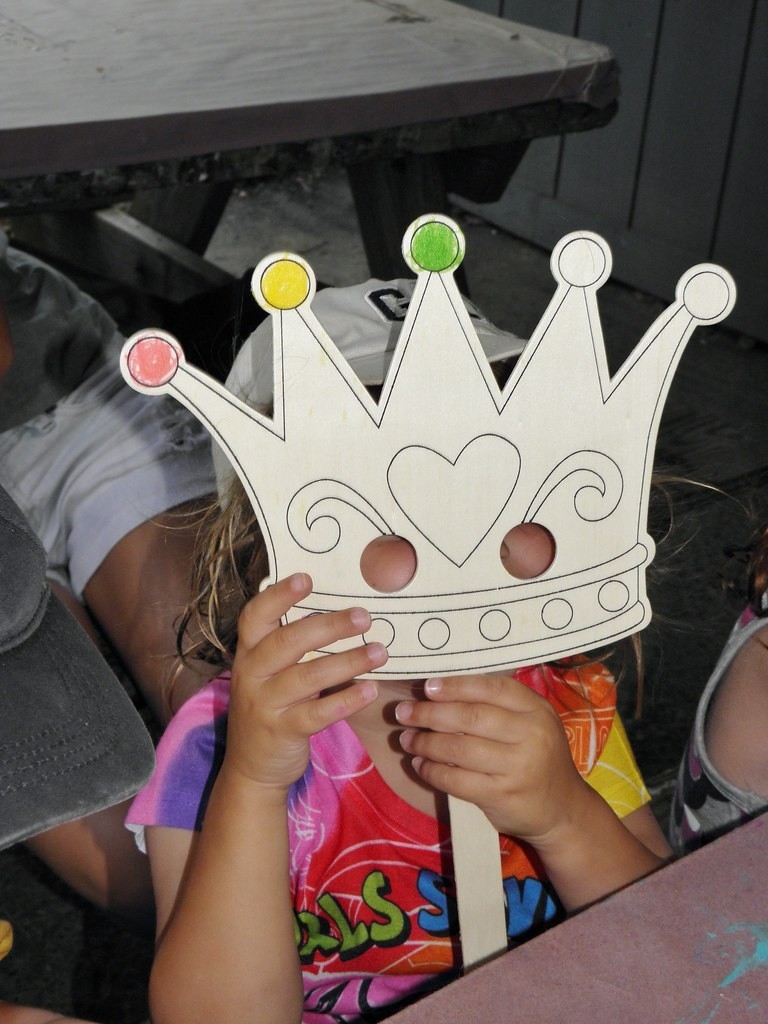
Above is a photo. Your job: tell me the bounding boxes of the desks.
[0,0,622,336]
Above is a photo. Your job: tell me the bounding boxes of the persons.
[669,515,768,856]
[0,239,249,1021]
[125,277,675,1024]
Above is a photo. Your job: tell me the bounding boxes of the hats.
[0,487,158,853]
[212,276,530,510]
